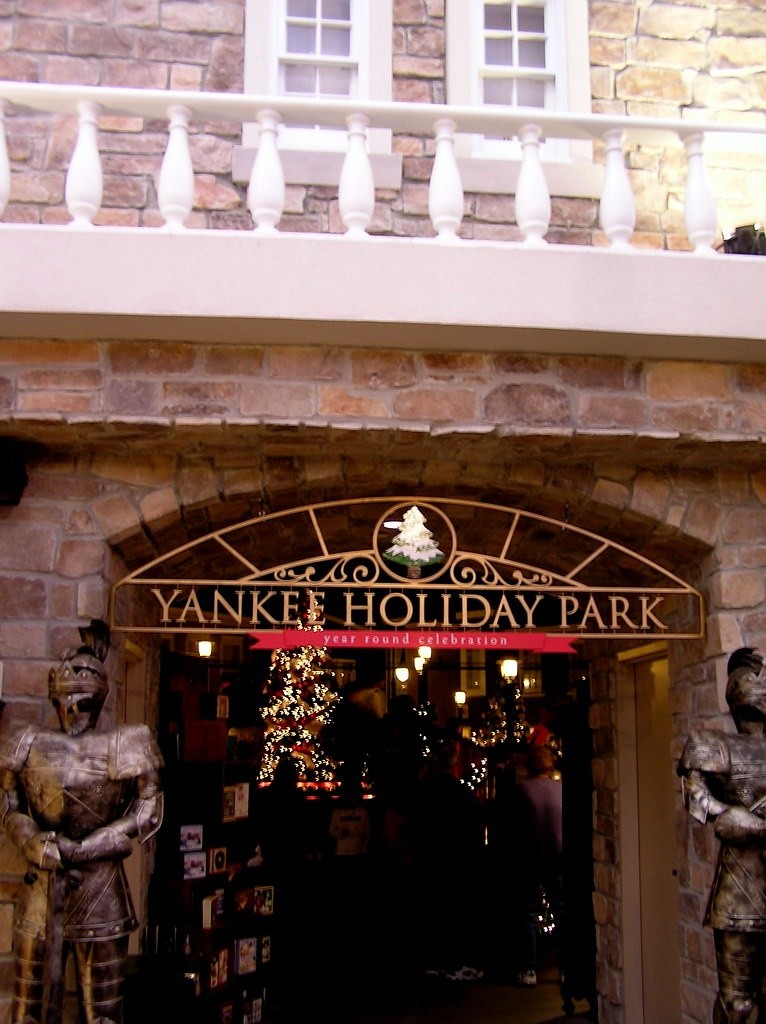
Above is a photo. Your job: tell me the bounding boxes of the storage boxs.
[203,896,218,929]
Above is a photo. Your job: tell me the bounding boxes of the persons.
[674,646,766,1024]
[327,695,563,985]
[1,617,166,1024]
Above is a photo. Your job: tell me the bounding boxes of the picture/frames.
[223,786,236,823]
[254,887,274,916]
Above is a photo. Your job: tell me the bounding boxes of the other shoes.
[445,966,485,982]
[516,969,537,986]
[427,964,446,976]
